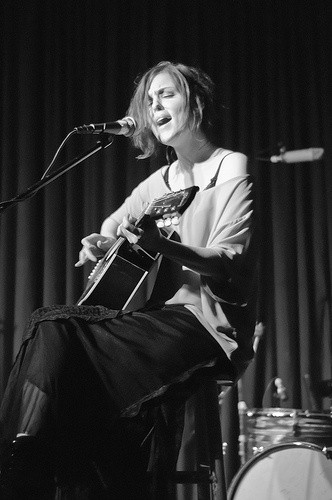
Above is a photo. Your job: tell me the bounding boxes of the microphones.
[71,116,137,139]
[267,144,324,164]
[251,321,264,354]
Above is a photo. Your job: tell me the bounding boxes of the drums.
[240,406,332,463]
[223,440,331,500]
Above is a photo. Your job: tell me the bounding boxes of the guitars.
[75,184,198,309]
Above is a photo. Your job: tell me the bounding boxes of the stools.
[140,362,239,500]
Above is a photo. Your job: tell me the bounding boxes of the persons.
[1,59,260,500]
